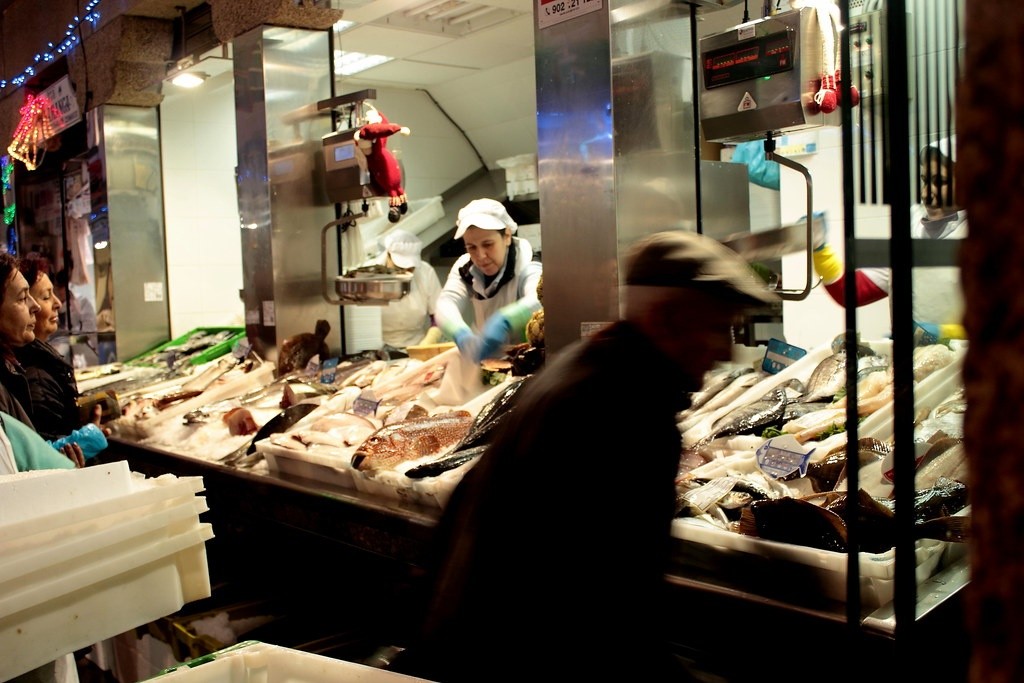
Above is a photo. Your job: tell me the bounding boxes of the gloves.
[454,315,511,361]
[913,319,938,346]
[796,212,827,252]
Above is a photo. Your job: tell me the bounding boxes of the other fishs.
[675,331,972,605]
[85,327,545,478]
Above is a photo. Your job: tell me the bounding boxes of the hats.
[384,229,424,269]
[624,230,780,312]
[921,133,957,166]
[453,196,518,240]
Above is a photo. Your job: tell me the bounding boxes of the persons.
[797,133,968,346]
[433,197,543,362]
[0,251,120,683]
[421,231,783,683]
[362,228,444,360]
